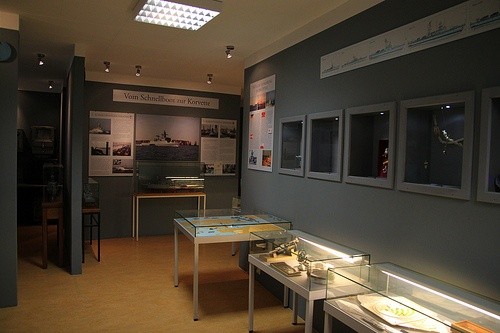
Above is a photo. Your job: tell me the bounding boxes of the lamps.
[37,54,45,67]
[48,82,53,89]
[104,62,110,73]
[224,45,234,59]
[135,66,141,77]
[129,0,222,32]
[207,74,213,84]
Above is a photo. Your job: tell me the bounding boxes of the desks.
[128,192,207,243]
